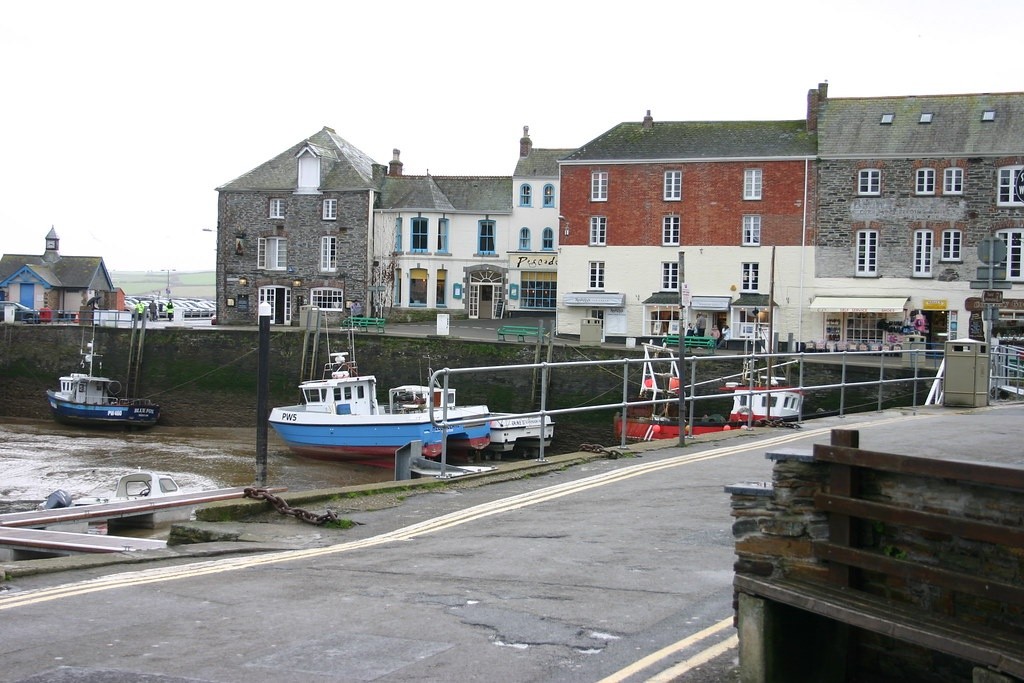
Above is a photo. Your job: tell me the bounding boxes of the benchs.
[342,317,386,333]
[497,325,548,343]
[662,334,717,355]
[992,359,1024,376]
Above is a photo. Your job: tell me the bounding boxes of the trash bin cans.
[435,314,450,335]
[173,307,184,326]
[579,317,603,347]
[901,334,927,368]
[4,305,15,325]
[299,305,320,330]
[943,338,990,408]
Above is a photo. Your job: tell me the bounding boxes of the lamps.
[291,280,301,289]
[239,278,250,288]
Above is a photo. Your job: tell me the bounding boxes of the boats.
[381,350,557,462]
[611,340,811,447]
[267,302,491,473]
[45,323,162,432]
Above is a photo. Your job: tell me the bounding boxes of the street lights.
[161,268,177,299]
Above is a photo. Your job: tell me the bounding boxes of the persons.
[686,312,732,350]
[351,299,358,317]
[94,300,100,309]
[135,299,174,321]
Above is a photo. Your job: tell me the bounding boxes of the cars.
[124,295,216,322]
[0,300,42,325]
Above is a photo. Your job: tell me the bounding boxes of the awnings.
[562,293,626,307]
[809,296,910,313]
[691,297,732,311]
[642,292,679,306]
[730,295,779,308]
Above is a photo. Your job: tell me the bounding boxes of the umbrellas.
[87,296,101,306]
